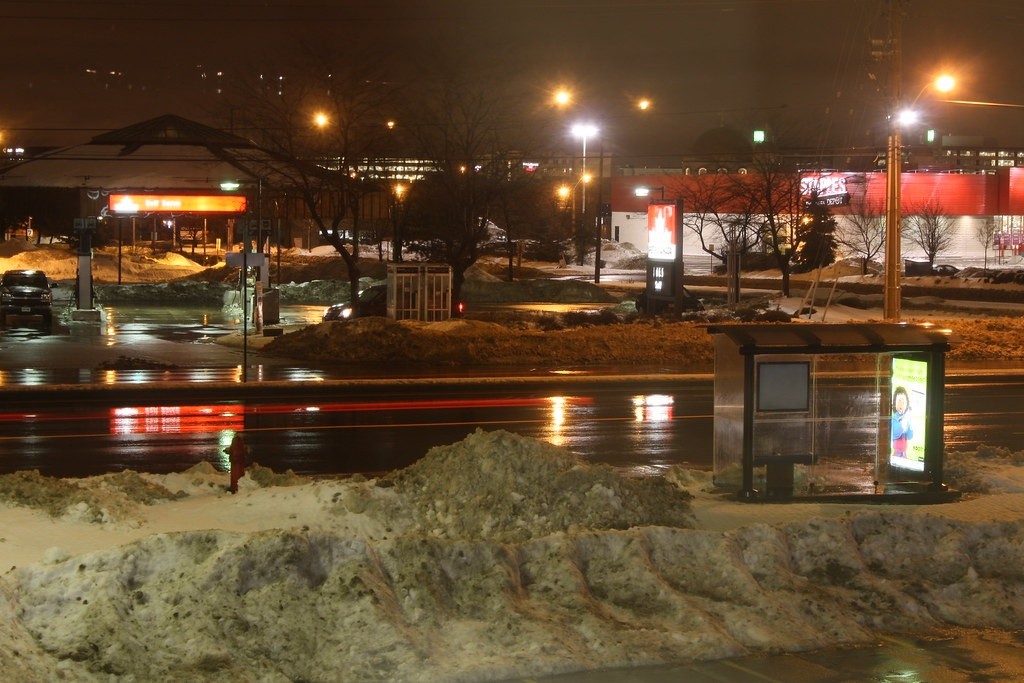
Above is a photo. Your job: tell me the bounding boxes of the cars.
[932,264,961,277]
[321,281,465,324]
[0,268,60,324]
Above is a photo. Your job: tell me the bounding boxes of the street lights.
[556,90,651,283]
[884,73,954,323]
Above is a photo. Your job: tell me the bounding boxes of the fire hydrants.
[223,434,249,493]
